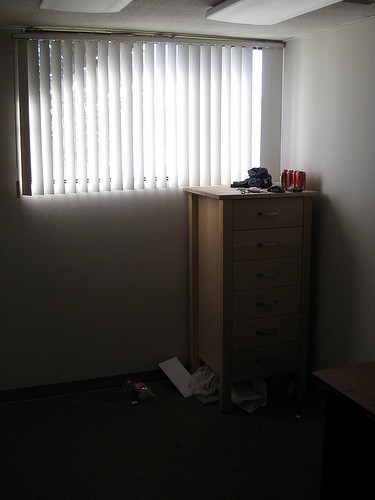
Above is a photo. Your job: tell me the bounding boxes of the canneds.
[296,171,307,190]
[281,169,288,189]
[293,170,298,187]
[286,169,293,188]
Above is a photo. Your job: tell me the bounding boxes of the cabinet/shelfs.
[312,361,375,500]
[184,186,321,414]
